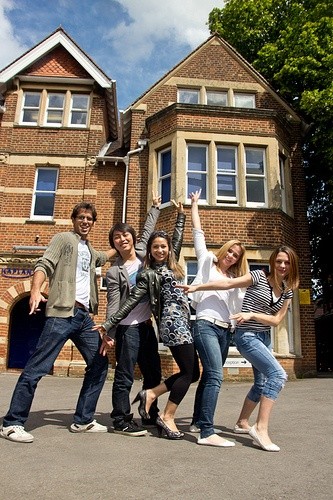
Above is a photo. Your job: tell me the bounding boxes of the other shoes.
[189,424,223,434]
[248,426,281,452]
[233,425,251,434]
[197,434,235,447]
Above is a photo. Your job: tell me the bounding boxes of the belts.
[75,301,84,309]
[196,315,229,328]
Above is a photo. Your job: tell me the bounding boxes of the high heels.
[132,389,151,420]
[154,416,184,440]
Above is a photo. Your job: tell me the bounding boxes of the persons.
[176,246,299,452]
[187,189,249,446]
[91,200,200,440]
[100,191,164,437]
[0,203,116,443]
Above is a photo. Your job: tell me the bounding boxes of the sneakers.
[70,419,115,432]
[113,413,148,436]
[0,423,34,443]
